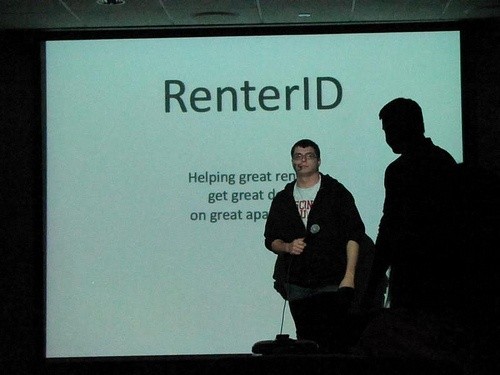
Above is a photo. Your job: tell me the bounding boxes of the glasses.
[293,152,317,160]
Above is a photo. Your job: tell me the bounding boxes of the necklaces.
[297,174,320,219]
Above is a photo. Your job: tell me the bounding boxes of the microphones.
[303,224,319,243]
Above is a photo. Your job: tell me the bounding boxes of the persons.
[264,140,366,352]
[371,97,460,306]
